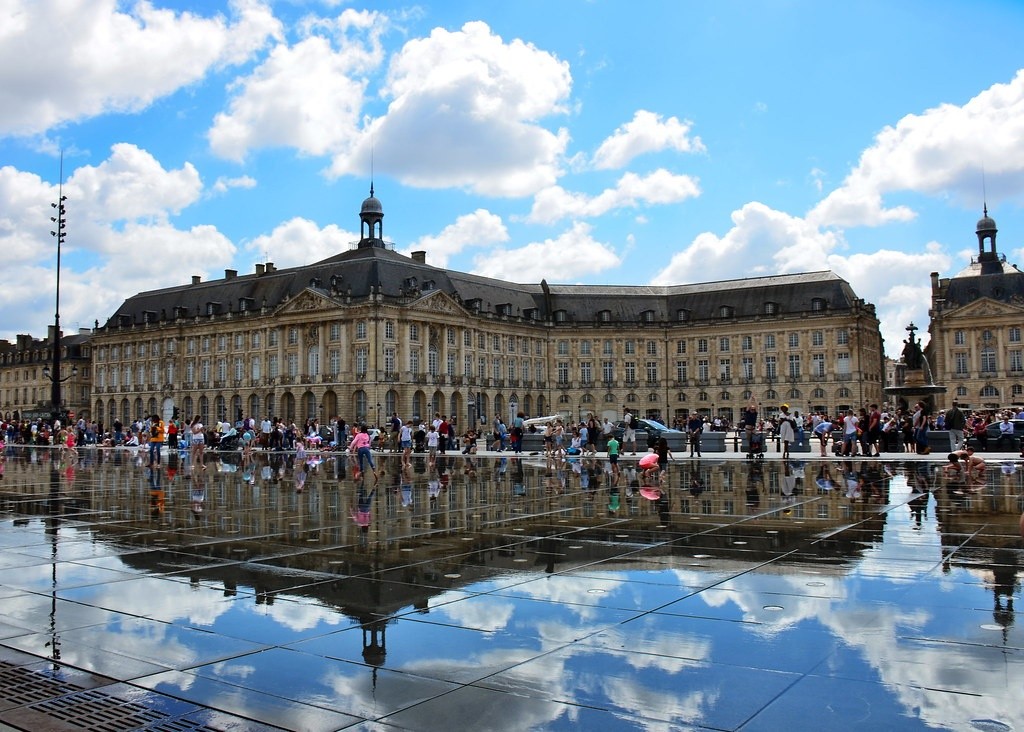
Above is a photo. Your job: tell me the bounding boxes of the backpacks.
[627,414,639,429]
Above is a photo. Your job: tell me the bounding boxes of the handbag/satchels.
[444,433,449,438]
[567,447,580,455]
[791,419,797,429]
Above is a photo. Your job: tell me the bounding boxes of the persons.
[189,469,206,520]
[350,480,377,533]
[945,475,987,496]
[471,395,935,458]
[1,412,479,478]
[942,447,986,475]
[688,461,933,503]
[493,458,527,496]
[1002,463,1016,477]
[377,456,480,507]
[930,397,1024,459]
[0,445,361,495]
[147,467,165,519]
[544,458,666,515]
[607,435,675,481]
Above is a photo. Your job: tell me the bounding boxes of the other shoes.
[591,450,597,456]
[497,450,502,452]
[851,453,855,457]
[873,453,880,457]
[586,450,589,456]
[621,451,624,455]
[504,447,508,451]
[145,463,154,467]
[921,452,929,455]
[630,453,636,455]
[515,452,522,455]
[690,453,694,457]
[374,471,377,477]
[866,453,872,457]
[491,446,493,451]
[156,465,161,468]
[201,465,207,471]
[698,455,701,457]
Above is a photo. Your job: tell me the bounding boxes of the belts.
[745,428,755,430]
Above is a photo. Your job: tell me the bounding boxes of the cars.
[611,419,683,435]
[964,418,1024,451]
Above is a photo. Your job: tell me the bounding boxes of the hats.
[780,404,789,410]
[37,418,42,420]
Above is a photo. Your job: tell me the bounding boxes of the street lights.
[759,402,762,423]
[319,404,324,428]
[807,400,812,419]
[511,401,515,426]
[44,363,78,419]
[269,406,271,419]
[472,403,476,430]
[378,402,381,427]
[712,403,714,420]
[428,403,431,426]
[578,404,581,423]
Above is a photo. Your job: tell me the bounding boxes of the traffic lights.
[238,408,243,420]
[173,406,180,420]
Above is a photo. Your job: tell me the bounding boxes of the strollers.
[746,427,765,459]
